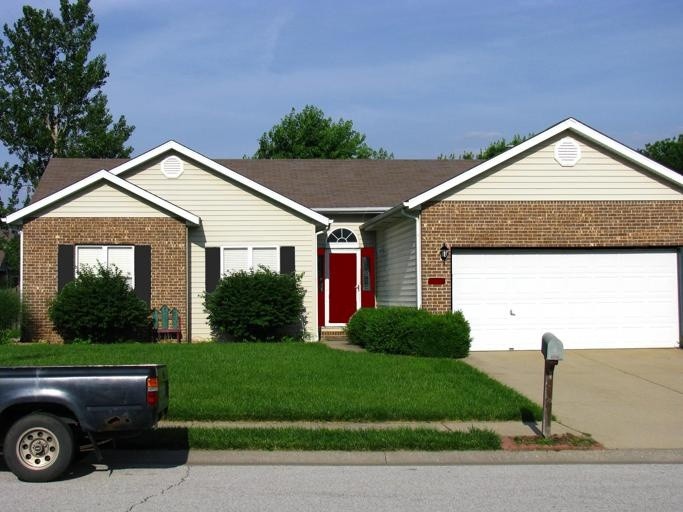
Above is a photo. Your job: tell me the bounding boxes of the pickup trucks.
[0,362,171,482]
